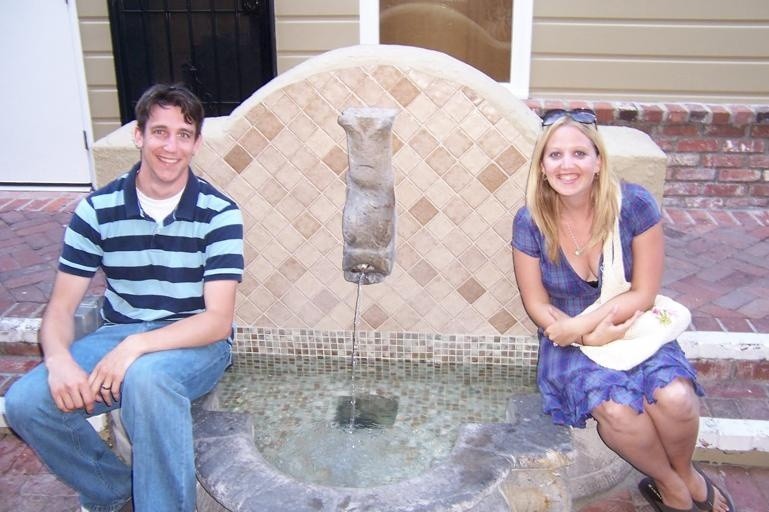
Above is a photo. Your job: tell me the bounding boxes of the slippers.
[638,467,733,512]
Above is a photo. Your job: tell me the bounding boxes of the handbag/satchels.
[574,283,692,372]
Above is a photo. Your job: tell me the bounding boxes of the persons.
[509,108,737,512]
[5,85,244,511]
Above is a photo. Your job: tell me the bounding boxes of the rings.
[552,342,559,348]
[98,384,112,391]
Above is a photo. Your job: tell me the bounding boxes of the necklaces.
[561,211,592,256]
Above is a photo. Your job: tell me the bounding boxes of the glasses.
[542,108,597,129]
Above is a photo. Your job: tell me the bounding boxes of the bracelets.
[579,334,587,347]
